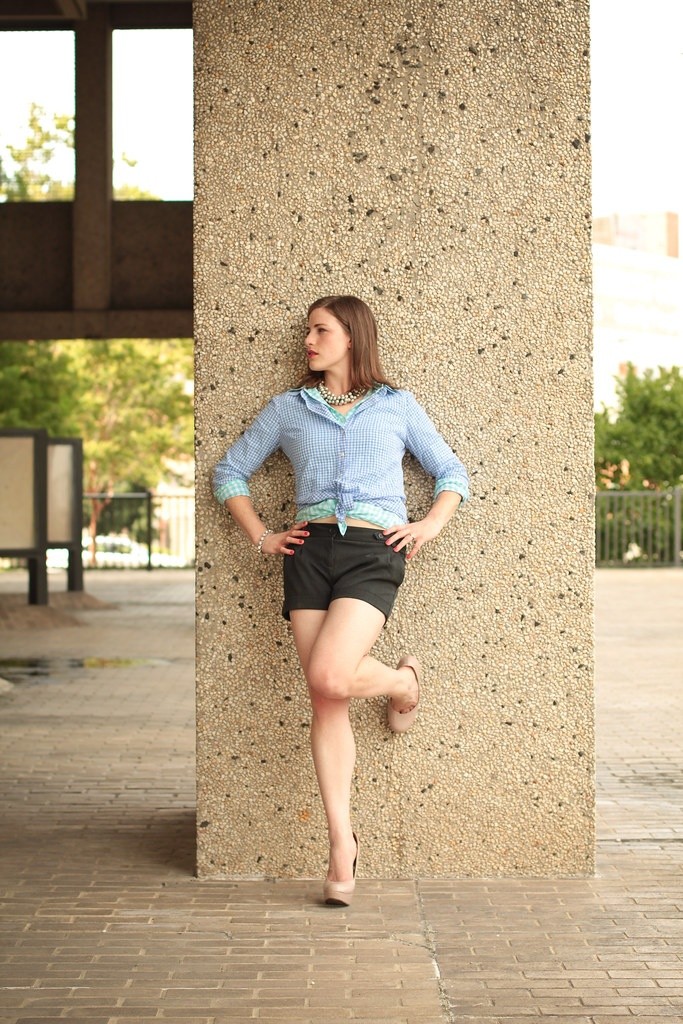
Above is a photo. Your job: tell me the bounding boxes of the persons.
[211,295,470,909]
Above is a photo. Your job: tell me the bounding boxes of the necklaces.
[317,377,367,406]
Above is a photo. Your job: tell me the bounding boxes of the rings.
[408,532,414,541]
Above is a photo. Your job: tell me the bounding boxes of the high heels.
[389,655,422,735]
[324,829,360,907]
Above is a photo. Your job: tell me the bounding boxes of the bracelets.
[257,529,274,556]
